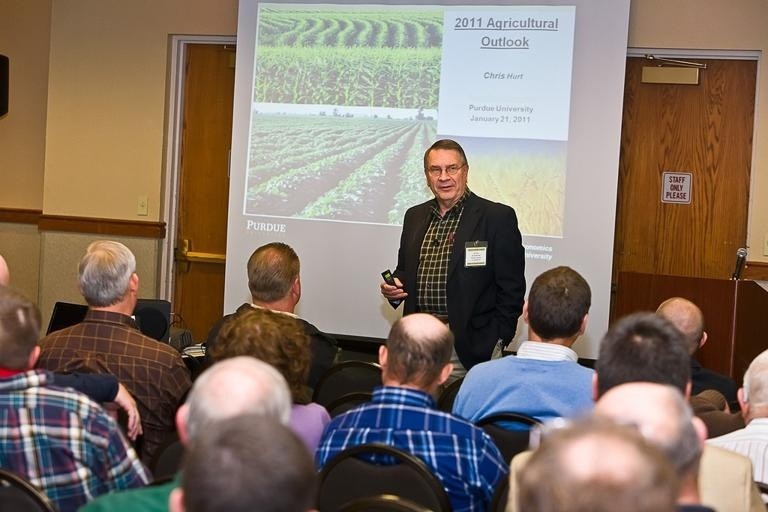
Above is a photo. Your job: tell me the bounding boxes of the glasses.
[426,165,465,175]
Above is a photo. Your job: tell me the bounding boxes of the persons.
[168,410,316,511]
[515,407,672,512]
[203,241,339,402]
[702,347,768,506]
[381,139,527,403]
[655,297,737,402]
[315,313,510,512]
[76,355,292,512]
[515,310,765,511]
[0,255,145,440]
[206,307,332,456]
[595,382,713,511]
[1,288,155,512]
[34,240,193,484]
[452,265,597,431]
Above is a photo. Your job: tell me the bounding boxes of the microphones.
[732,247,747,280]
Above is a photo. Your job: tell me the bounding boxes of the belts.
[437,317,449,324]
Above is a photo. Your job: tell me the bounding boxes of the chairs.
[475,411,545,465]
[0,470,51,512]
[326,391,373,419]
[312,441,452,512]
[309,360,383,402]
[329,493,431,512]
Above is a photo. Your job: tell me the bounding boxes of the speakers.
[45,301,89,336]
[133,299,171,344]
[0,54,9,116]
